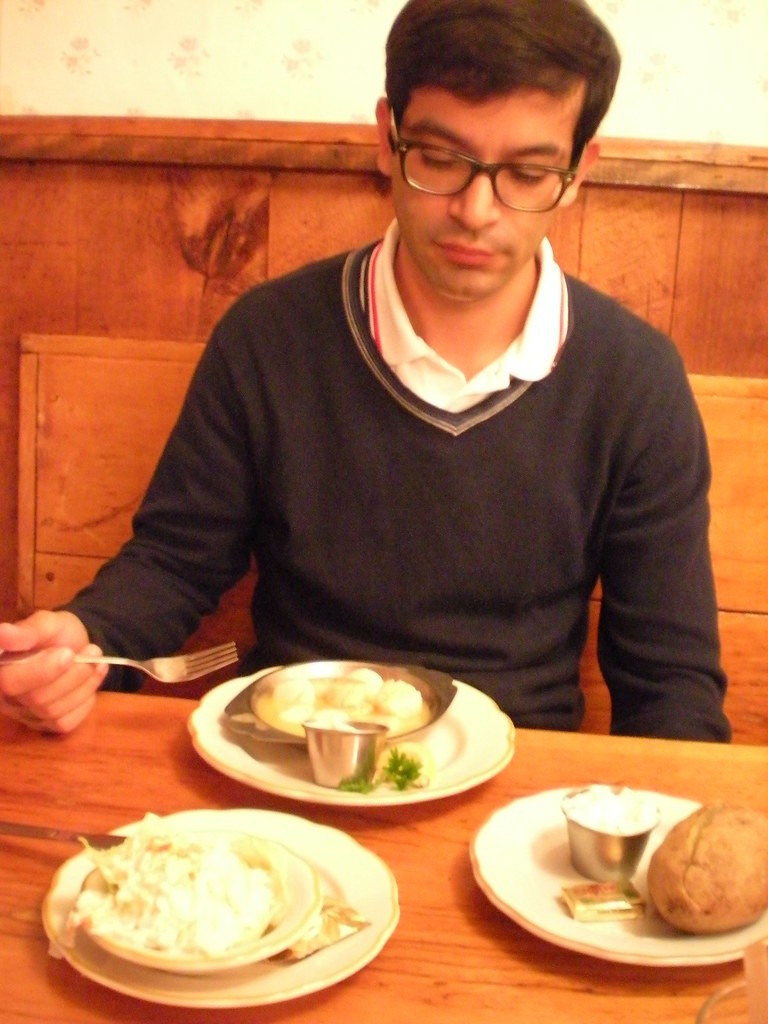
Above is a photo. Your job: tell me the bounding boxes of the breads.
[646,801,768,934]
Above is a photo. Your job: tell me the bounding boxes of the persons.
[0,2,732,745]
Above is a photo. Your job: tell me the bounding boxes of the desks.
[2,692,767,1021]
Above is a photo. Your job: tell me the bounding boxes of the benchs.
[16,329,768,749]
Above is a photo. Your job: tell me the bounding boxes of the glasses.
[385,106,574,213]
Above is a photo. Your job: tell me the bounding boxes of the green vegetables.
[340,747,425,792]
